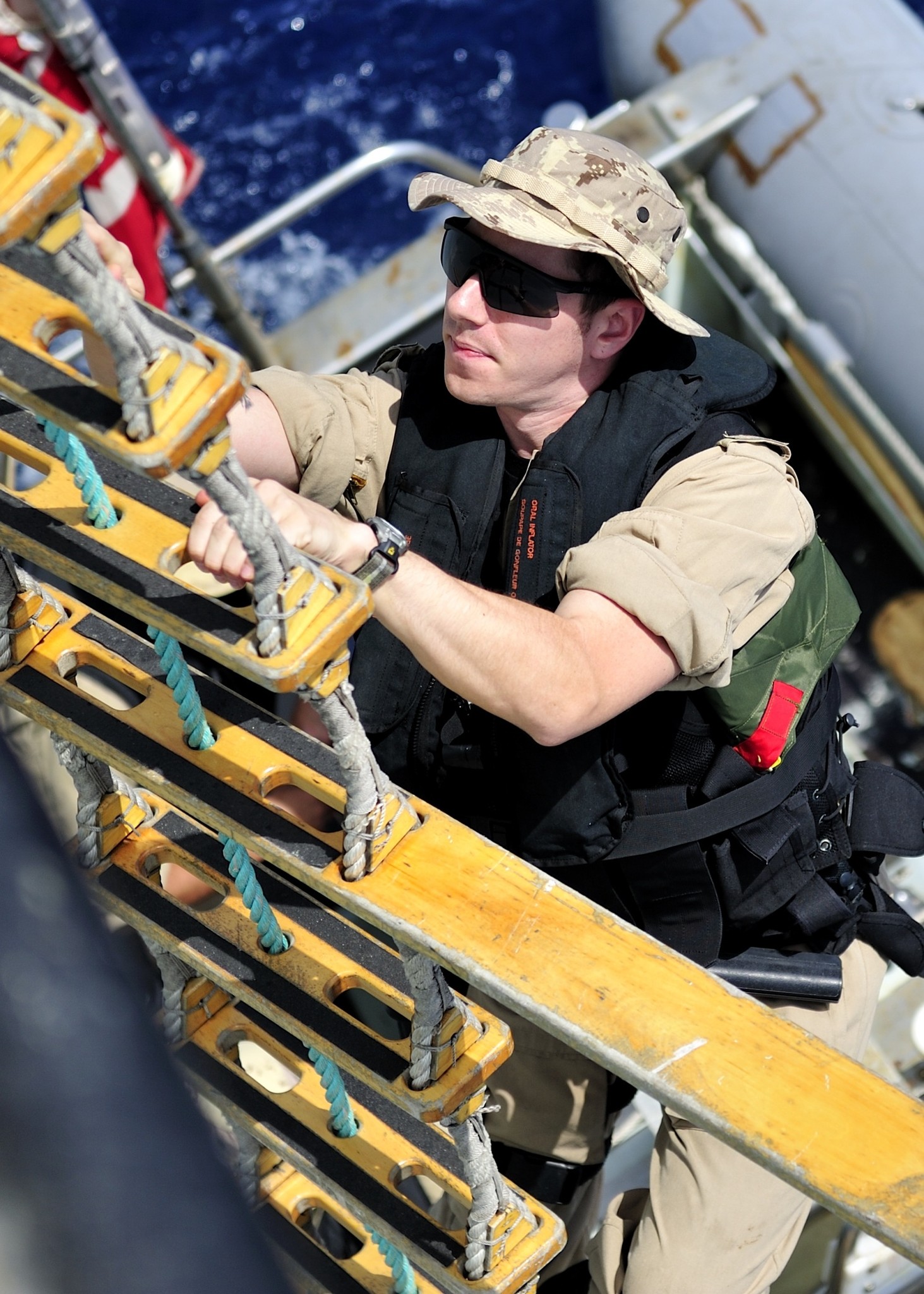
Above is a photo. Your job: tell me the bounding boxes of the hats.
[406,124,712,338]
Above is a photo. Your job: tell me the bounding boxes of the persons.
[161,125,890,1293]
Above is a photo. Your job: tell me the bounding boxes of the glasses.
[439,216,619,319]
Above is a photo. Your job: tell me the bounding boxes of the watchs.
[348,516,408,591]
